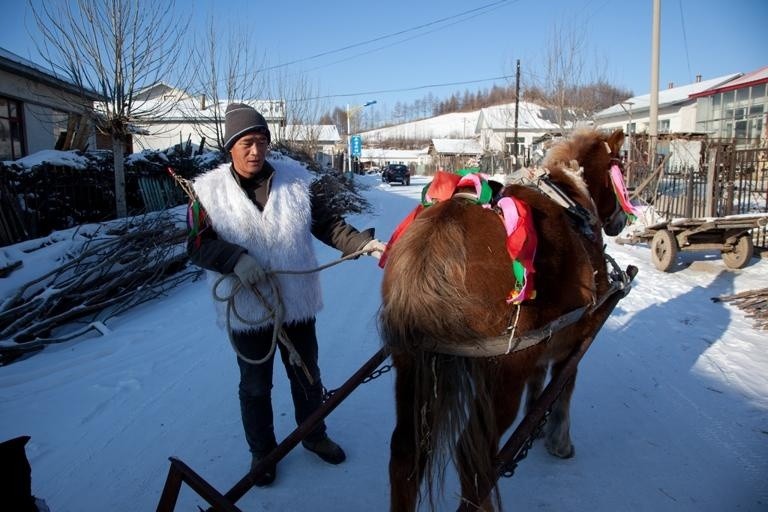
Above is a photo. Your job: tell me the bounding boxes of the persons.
[183,100,392,490]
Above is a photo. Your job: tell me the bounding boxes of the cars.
[366,166,382,176]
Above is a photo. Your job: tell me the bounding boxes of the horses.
[374,124,628,511]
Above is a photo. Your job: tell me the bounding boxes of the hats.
[221,101,271,152]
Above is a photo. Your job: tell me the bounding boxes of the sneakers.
[301,433,347,468]
[249,444,280,489]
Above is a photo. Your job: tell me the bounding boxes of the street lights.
[346,100,378,180]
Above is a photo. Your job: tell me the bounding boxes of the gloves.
[361,238,390,260]
[233,252,268,295]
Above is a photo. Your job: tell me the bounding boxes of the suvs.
[380,162,411,185]
